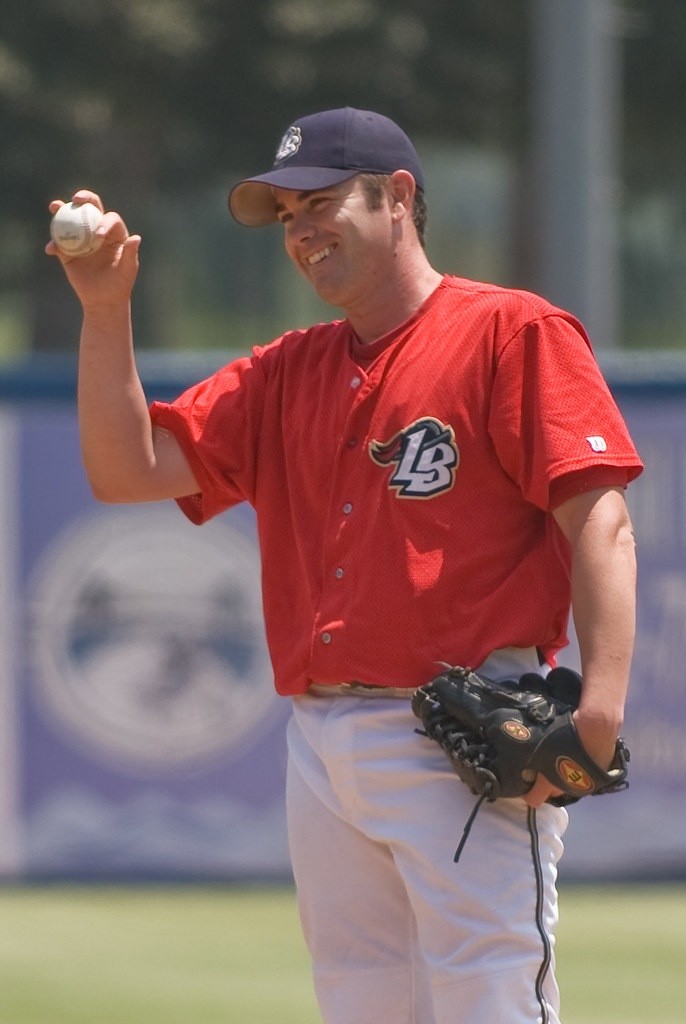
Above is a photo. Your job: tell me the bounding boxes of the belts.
[535,646,547,666]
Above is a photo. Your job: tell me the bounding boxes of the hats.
[227,106,425,227]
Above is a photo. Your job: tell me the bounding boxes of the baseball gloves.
[410,666,632,808]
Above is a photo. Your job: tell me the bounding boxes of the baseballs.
[49,202,104,259]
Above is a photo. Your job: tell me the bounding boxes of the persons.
[44,106,643,1024]
